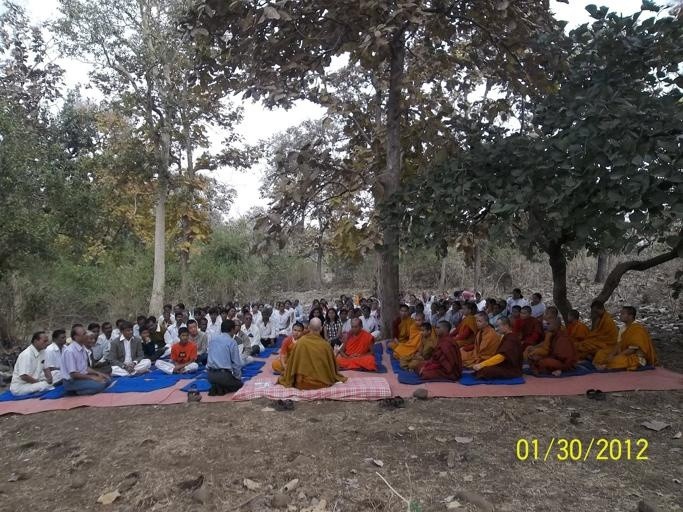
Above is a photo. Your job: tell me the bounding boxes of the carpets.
[0,322,682,413]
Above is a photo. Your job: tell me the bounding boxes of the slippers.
[587,389,603,400]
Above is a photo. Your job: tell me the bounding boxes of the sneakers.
[275,399,293,411]
[383,397,405,409]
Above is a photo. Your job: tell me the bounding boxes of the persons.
[10,330,61,399]
[43,301,280,387]
[387,289,658,383]
[273,293,384,389]
[206,319,243,397]
